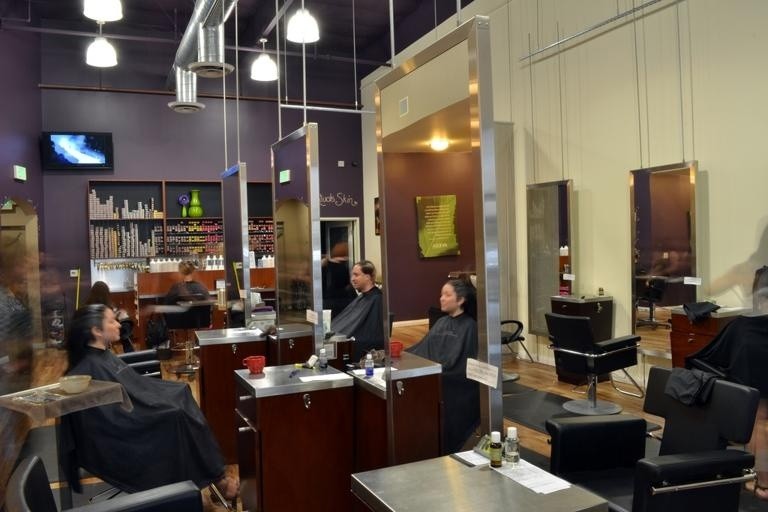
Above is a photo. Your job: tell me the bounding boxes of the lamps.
[286,0,319,44]
[250,39,278,83]
[83,20,118,68]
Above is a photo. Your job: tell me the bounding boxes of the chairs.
[116,349,162,380]
[4,453,203,511]
[110,318,135,354]
[544,365,759,511]
[545,312,645,416]
[502,320,534,363]
[87,482,238,511]
[389,312,396,337]
[502,372,520,382]
[165,291,215,380]
[429,305,448,330]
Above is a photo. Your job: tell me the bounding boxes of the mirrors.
[627,158,698,367]
[371,10,504,457]
[219,162,252,327]
[268,116,326,365]
[521,174,569,341]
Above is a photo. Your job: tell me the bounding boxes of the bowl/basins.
[59,375,92,394]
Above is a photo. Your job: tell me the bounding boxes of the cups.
[241,355,267,375]
[390,341,403,358]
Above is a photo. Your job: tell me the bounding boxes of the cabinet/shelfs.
[552,296,613,340]
[347,349,442,467]
[233,363,354,511]
[108,291,138,344]
[4,379,134,427]
[349,474,394,512]
[84,176,270,261]
[194,328,266,465]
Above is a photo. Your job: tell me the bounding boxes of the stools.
[636,275,672,330]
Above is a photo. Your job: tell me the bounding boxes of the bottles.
[148,257,183,273]
[262,254,274,268]
[318,348,329,372]
[488,430,503,467]
[205,255,224,271]
[505,426,520,468]
[364,353,374,378]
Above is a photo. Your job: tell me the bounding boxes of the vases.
[182,205,187,217]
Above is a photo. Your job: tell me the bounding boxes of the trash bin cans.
[147,320,171,360]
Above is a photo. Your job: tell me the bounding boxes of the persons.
[84,280,138,350]
[400,278,479,454]
[145,259,213,346]
[66,308,240,503]
[332,260,383,362]
[322,243,351,311]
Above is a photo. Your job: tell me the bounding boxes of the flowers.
[178,194,189,205]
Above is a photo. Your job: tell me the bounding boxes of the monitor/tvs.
[40,130,113,169]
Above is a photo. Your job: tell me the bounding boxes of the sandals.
[210,477,241,504]
